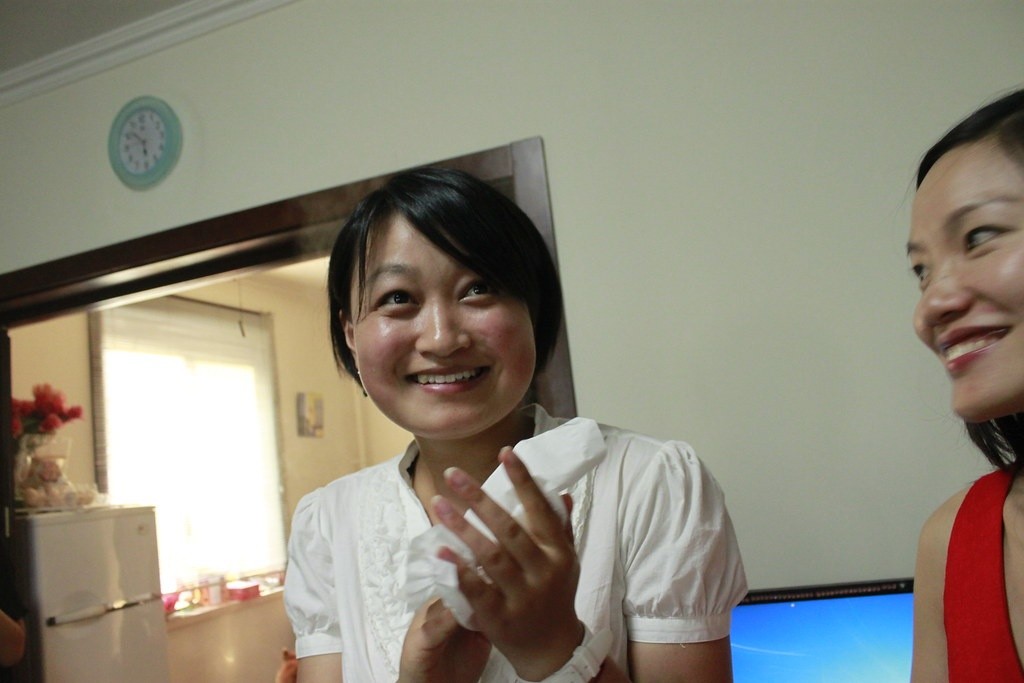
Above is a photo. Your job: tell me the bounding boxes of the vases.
[13,433,73,506]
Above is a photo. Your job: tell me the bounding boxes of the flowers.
[9,382,82,439]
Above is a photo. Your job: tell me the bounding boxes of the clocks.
[107,95,182,190]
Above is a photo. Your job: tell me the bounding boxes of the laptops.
[725,577,914,683]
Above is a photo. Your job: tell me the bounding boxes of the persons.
[281,164,749,683]
[904,88,1024,683]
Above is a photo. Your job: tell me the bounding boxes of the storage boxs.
[228,582,259,599]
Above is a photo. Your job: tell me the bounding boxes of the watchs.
[534,621,616,682]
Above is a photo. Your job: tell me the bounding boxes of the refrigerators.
[14,501,173,683]
